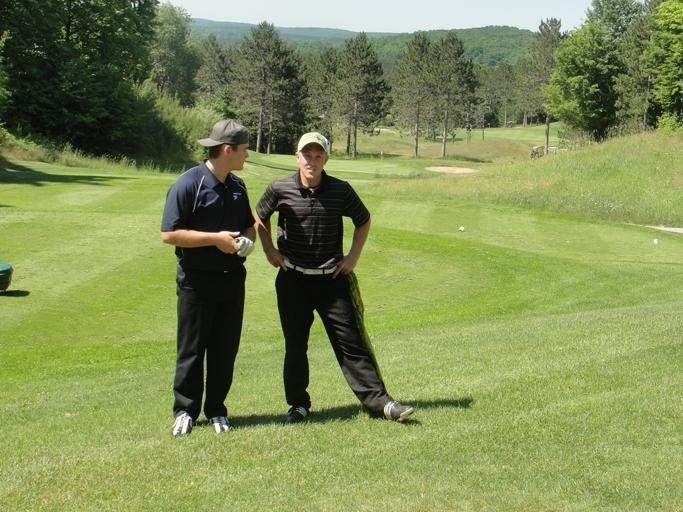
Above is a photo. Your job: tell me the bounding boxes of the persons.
[252,131,416,424]
[159,117,257,439]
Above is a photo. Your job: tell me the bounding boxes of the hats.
[197,119,250,147]
[298,132,330,155]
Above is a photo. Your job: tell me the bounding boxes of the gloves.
[234,236,255,258]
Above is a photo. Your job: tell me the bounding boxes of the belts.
[283,259,338,275]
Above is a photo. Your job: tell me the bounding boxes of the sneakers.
[380,399,414,421]
[286,405,311,422]
[171,412,193,437]
[208,415,235,433]
